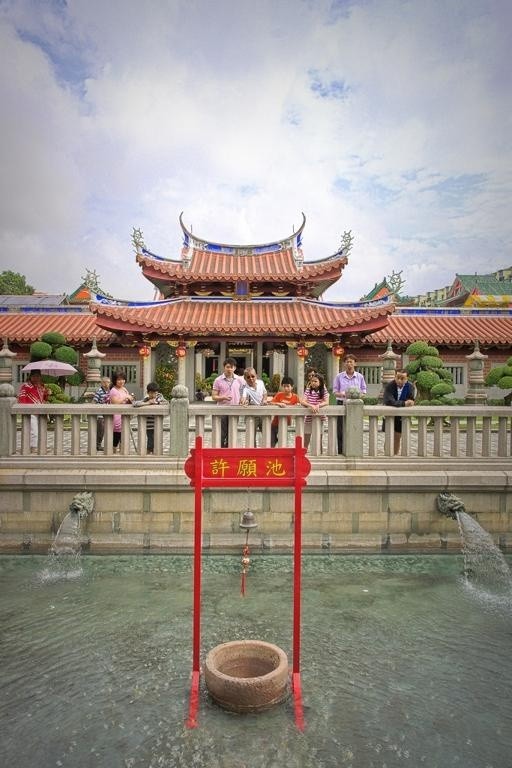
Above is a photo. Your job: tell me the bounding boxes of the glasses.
[246,374,255,379]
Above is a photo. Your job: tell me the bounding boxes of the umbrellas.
[19,358,79,377]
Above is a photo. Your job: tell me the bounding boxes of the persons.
[304,367,325,388]
[18,369,55,454]
[133,380,170,456]
[300,375,328,455]
[331,354,366,456]
[105,371,135,454]
[213,357,246,451]
[380,369,418,456]
[270,377,300,448]
[239,365,270,450]
[90,375,113,452]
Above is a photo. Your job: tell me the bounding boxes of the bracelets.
[319,404,322,407]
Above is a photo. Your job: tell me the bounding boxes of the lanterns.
[332,345,348,358]
[174,343,187,361]
[297,345,312,359]
[138,345,152,362]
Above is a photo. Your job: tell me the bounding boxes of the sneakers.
[97,447,105,451]
